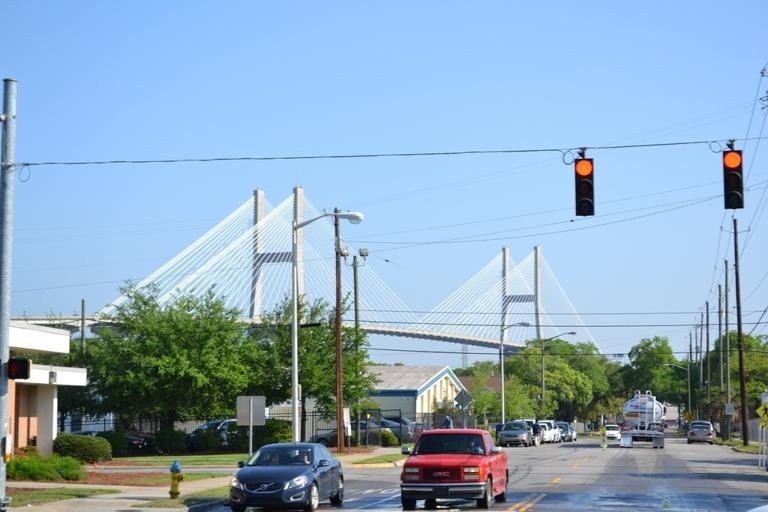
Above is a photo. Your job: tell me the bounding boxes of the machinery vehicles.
[617,390,666,448]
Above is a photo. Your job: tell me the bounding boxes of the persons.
[464,437,479,454]
[296,452,311,464]
[440,413,454,428]
[532,418,541,448]
[268,452,281,465]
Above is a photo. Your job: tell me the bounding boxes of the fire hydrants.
[169,461,185,499]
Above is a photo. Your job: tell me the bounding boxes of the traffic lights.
[9,359,30,379]
[574,157,594,217]
[723,145,744,210]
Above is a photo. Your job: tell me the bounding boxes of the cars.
[400,428,509,511]
[306,419,387,447]
[688,425,715,445]
[687,421,716,440]
[605,424,621,440]
[72,430,155,457]
[188,418,239,445]
[367,417,412,445]
[499,418,578,447]
[230,444,346,510]
[382,416,430,446]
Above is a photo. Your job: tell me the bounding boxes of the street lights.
[291,208,363,445]
[541,330,576,419]
[498,320,531,425]
